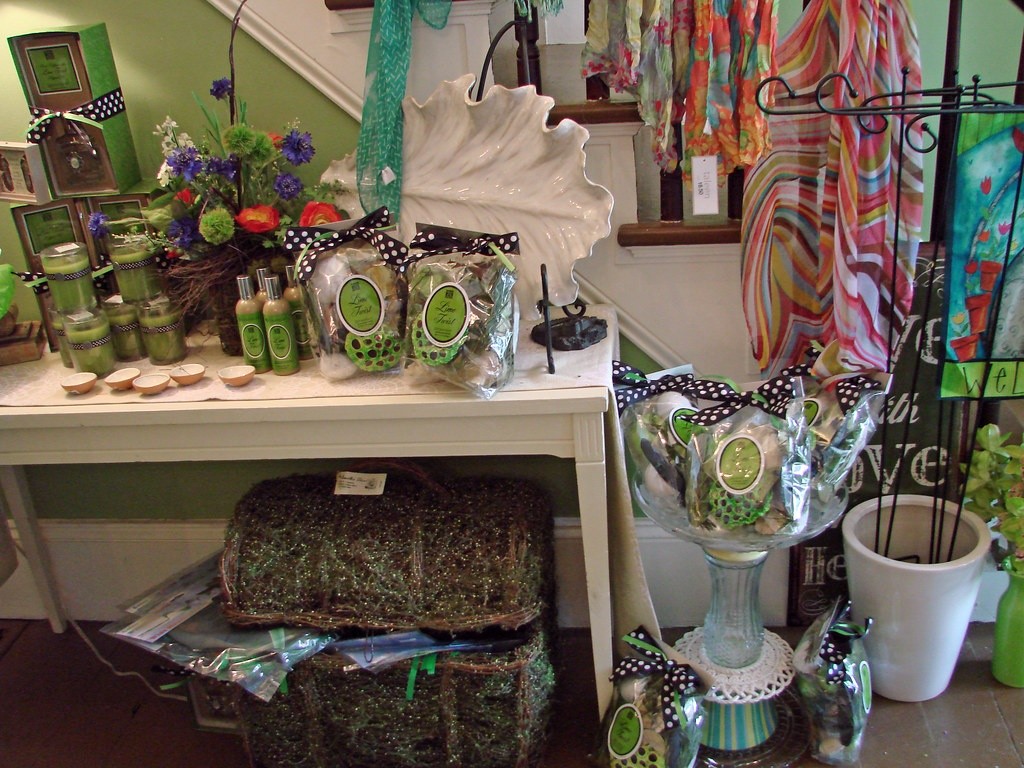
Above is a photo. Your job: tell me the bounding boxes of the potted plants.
[958,423,1024,689]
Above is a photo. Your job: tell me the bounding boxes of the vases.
[212,243,294,355]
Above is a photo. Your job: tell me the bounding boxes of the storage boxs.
[87,172,166,272]
[6,22,142,198]
[9,198,96,354]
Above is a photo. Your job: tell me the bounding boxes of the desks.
[0,299,662,723]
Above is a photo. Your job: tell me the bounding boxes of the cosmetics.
[39,240,189,378]
[232,263,315,376]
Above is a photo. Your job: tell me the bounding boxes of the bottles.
[235,264,320,376]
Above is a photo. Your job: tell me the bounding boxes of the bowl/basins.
[104,367,141,390]
[60,372,97,395]
[132,373,171,395]
[218,365,256,386]
[169,363,205,385]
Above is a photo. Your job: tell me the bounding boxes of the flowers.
[88,76,353,249]
